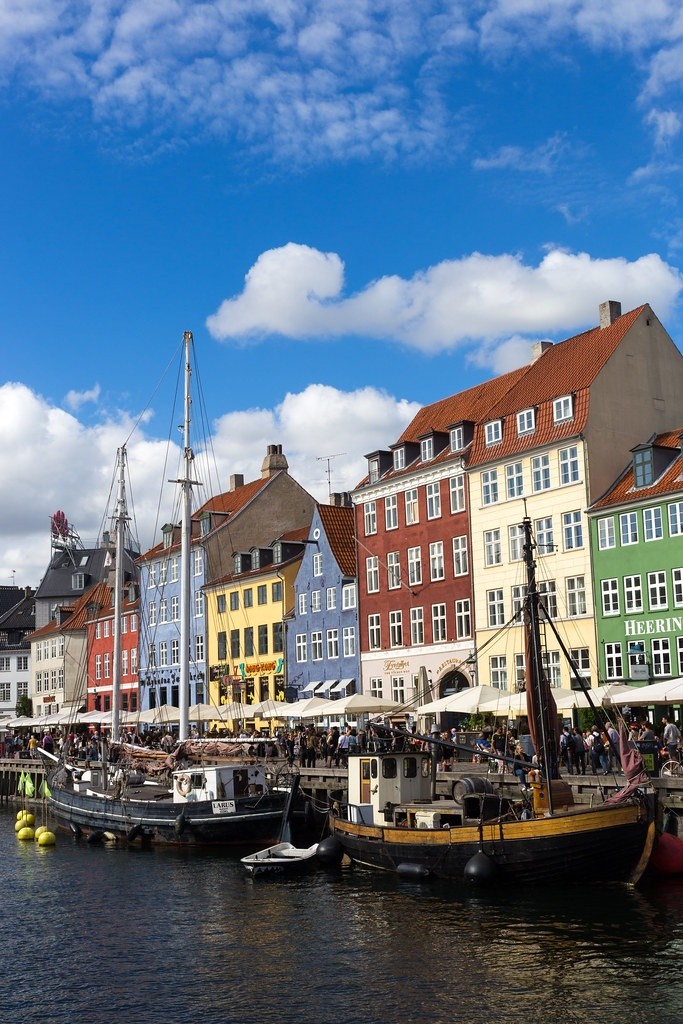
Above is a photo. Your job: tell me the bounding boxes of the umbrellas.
[0,690,416,733]
[419,676,683,717]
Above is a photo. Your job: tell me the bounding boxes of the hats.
[127,731,132,735]
[191,725,196,728]
[628,722,639,729]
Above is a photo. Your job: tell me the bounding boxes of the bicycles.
[659,743,683,777]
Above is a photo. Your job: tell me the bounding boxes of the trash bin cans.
[520,735,536,756]
[78,741,86,758]
[635,740,659,777]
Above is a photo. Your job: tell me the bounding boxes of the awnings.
[330,677,357,693]
[302,680,322,695]
[315,678,339,695]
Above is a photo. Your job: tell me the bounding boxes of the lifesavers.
[127,826,151,846]
[176,774,191,796]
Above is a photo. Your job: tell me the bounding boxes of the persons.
[3,717,682,772]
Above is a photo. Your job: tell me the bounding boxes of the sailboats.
[32,329,310,848]
[327,496,662,897]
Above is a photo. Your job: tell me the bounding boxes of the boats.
[240,840,321,879]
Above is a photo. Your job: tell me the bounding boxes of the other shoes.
[526,787,534,791]
[566,764,625,776]
[522,785,527,792]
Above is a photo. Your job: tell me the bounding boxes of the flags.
[25,772,35,797]
[17,769,25,794]
[39,779,52,800]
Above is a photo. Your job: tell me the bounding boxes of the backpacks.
[562,732,576,750]
[591,732,604,751]
